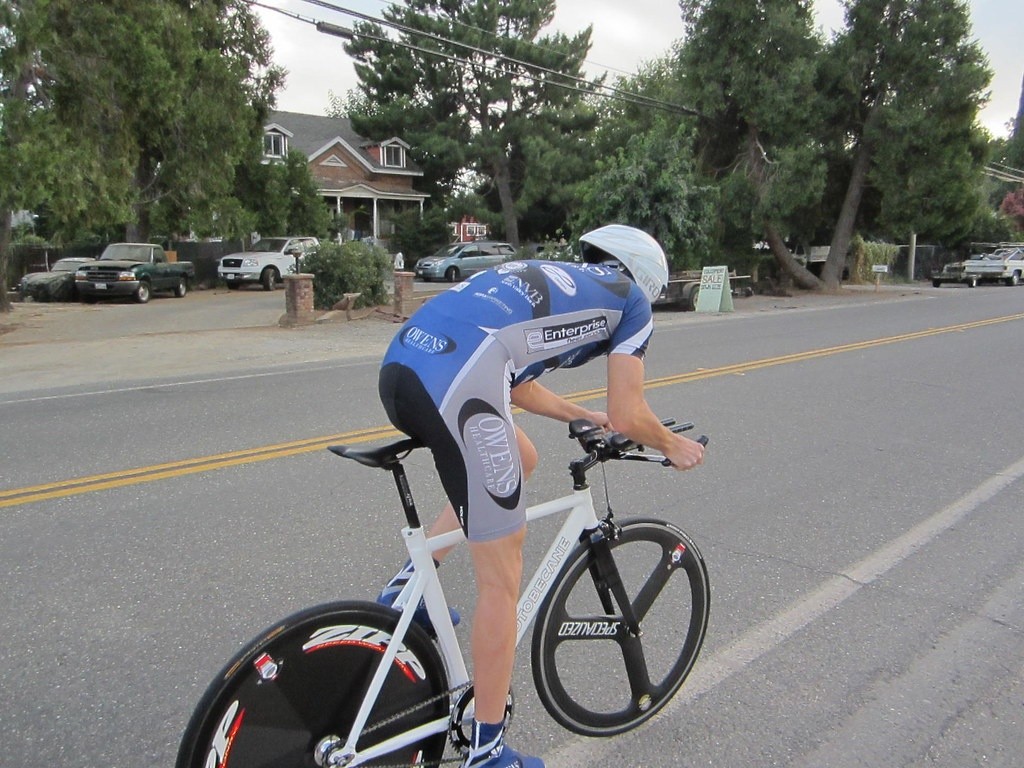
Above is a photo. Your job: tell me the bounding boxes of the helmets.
[577,219,672,306]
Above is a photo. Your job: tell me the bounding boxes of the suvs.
[218,238,319,292]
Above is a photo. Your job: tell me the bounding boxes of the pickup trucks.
[75,241,193,303]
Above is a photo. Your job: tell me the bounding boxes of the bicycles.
[178,415,716,768]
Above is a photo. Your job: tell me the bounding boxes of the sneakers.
[378,552,460,627]
[459,715,548,768]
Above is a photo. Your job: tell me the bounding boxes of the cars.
[927,245,1024,289]
[415,241,517,281]
[17,257,96,302]
[653,268,738,311]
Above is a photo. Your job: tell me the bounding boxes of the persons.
[378,223,703,768]
